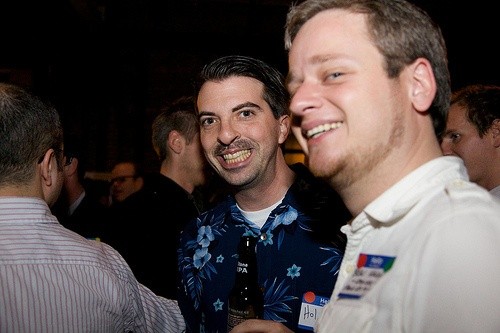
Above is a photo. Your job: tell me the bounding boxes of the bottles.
[227,239,264,333]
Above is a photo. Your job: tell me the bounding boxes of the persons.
[0,82,186,333]
[48,136,109,243]
[440,85,500,201]
[157,56,353,333]
[101,95,211,299]
[229,0,500,333]
[108,158,145,204]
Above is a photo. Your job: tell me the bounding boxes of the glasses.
[110,176,134,183]
[37,145,73,166]
